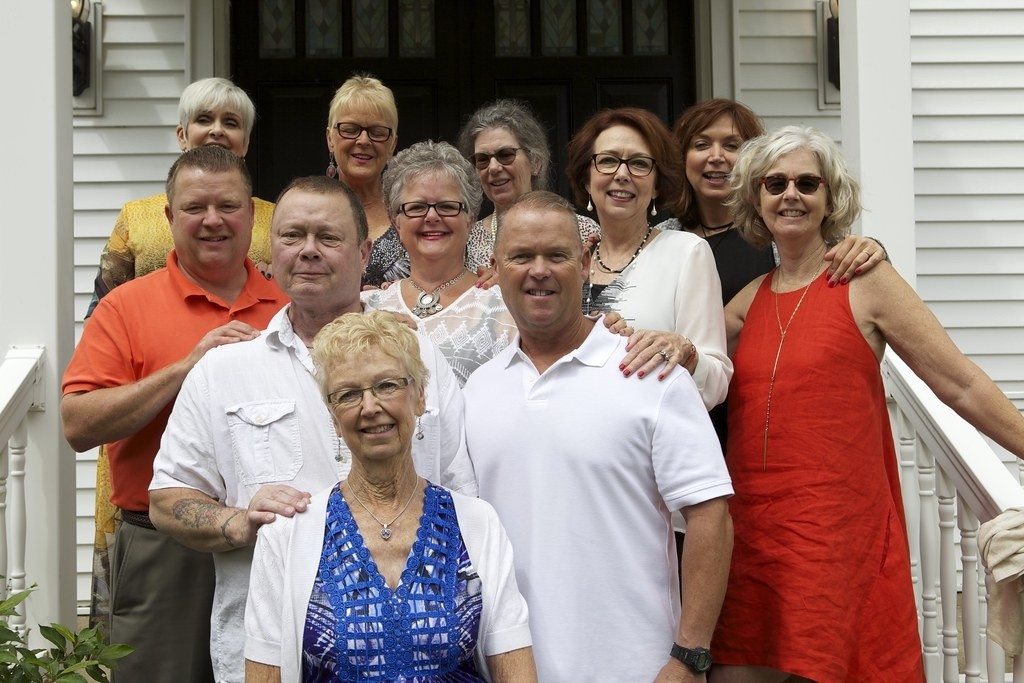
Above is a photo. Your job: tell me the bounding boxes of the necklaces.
[698,221,734,230]
[406,266,467,319]
[491,207,498,247]
[346,471,419,540]
[585,220,653,315]
[762,246,828,469]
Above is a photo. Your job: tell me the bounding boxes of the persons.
[722,124,1024,683]
[244,311,537,683]
[358,139,520,386]
[654,97,890,306]
[148,174,480,682]
[80,77,276,683]
[325,75,410,288]
[459,190,734,683]
[59,144,289,683]
[564,105,734,413]
[458,99,602,277]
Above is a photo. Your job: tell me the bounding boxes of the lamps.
[828,0,840,91]
[69,0,92,94]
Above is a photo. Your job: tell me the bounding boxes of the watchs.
[670,642,714,675]
[684,336,696,368]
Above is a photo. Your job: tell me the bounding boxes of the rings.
[659,350,669,361]
[862,250,870,256]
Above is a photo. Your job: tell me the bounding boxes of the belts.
[120,508,158,531]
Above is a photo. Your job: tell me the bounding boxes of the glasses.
[334,121,394,142]
[756,172,829,195]
[395,200,470,218]
[327,375,414,410]
[588,152,659,178]
[462,146,527,171]
